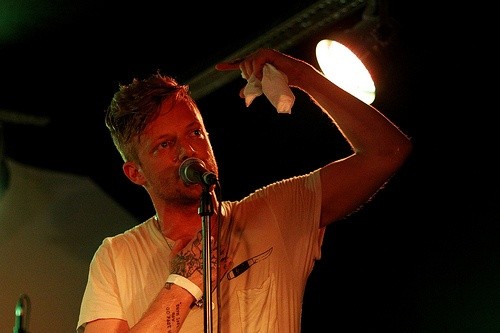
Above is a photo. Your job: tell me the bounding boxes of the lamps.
[316,24,395,105]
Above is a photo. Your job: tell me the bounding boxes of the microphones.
[179,158,217,185]
[13,295,25,333]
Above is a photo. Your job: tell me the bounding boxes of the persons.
[77,46,412,333]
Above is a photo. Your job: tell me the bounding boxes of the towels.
[238,60,296,115]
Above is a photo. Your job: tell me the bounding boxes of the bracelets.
[164,274,204,303]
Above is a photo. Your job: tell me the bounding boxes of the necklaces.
[154,214,217,253]
